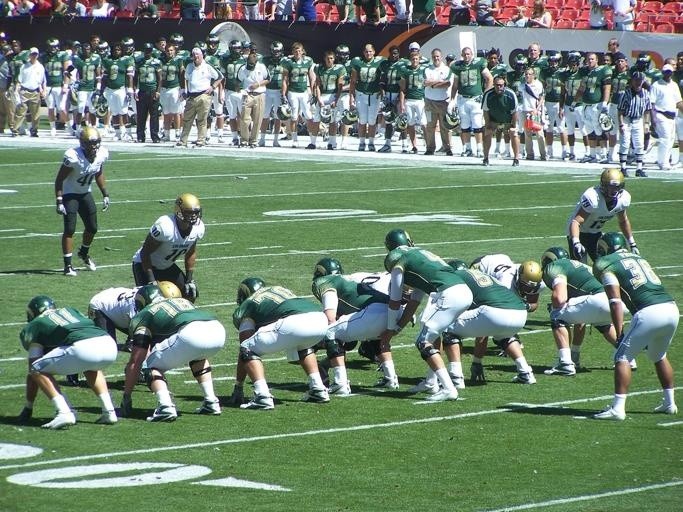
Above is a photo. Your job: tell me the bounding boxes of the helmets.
[602,169,625,202]
[175,193,202,226]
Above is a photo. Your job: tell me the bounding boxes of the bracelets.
[472,354,484,365]
[571,344,582,353]
[56,197,63,204]
[571,237,581,245]
[24,399,33,409]
[235,380,244,387]
[627,236,635,244]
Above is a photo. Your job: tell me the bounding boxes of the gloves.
[629,237,640,254]
[102,195,110,212]
[56,196,66,214]
[4,408,32,425]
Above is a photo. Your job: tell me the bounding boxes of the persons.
[1,0,639,31]
[55,127,110,276]
[19,296,118,429]
[67,281,183,386]
[567,169,640,266]
[539,247,638,376]
[468,253,545,357]
[121,285,227,422]
[124,193,205,352]
[480,38,682,177]
[385,230,473,401]
[441,260,536,390]
[231,278,330,410]
[312,258,404,395]
[1,34,494,158]
[593,232,680,420]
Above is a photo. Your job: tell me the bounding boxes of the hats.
[662,64,673,73]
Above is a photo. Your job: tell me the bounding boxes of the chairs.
[425,0,682,33]
